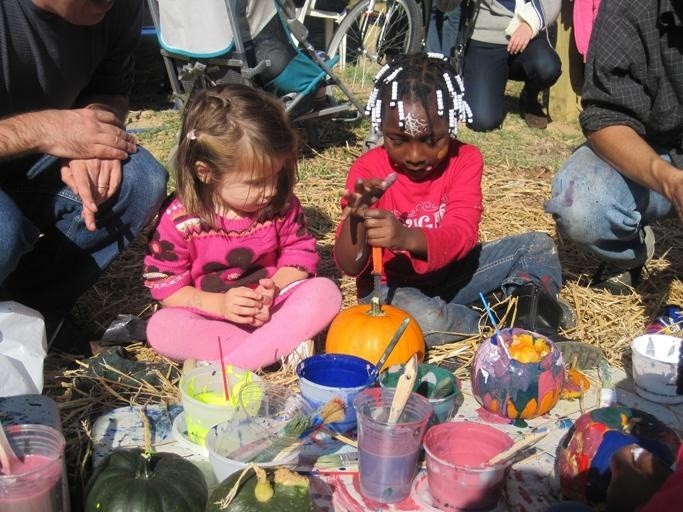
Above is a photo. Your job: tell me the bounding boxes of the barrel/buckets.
[178,353,517,512]
[630,333,683,405]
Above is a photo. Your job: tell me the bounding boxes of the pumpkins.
[206,463,314,512]
[325,297,426,374]
[83,448,208,512]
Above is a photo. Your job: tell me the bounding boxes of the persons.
[143,84,343,373]
[332,52,575,351]
[544,0,683,296]
[463,0,562,132]
[0,0,170,358]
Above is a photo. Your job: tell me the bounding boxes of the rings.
[97,184,109,189]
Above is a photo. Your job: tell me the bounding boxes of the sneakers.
[588,244,657,298]
[519,89,548,130]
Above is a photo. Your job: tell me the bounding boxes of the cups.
[218,381,315,464]
[352,387,434,503]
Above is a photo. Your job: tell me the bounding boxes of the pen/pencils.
[218,336,230,406]
[480,292,512,360]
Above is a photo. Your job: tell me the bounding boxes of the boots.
[487,284,603,369]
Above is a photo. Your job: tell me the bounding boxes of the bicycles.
[276,0,427,125]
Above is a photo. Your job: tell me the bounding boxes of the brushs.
[226,396,346,462]
